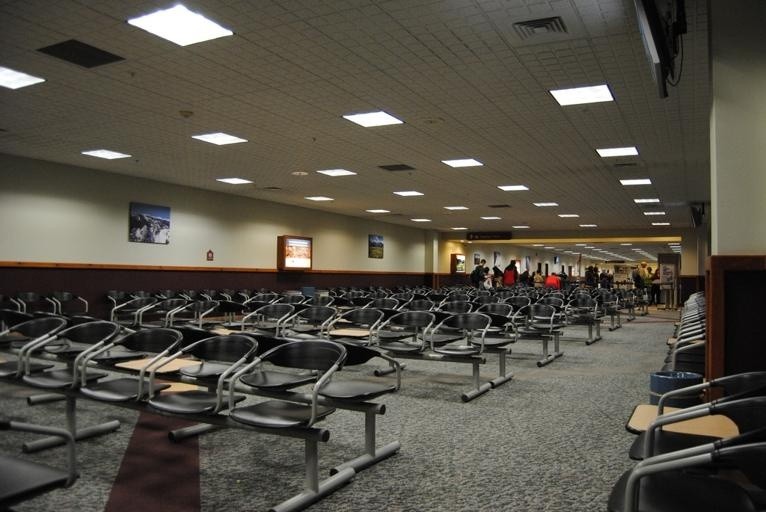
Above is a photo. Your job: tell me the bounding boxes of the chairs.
[0,286,656,511]
[606,290,765,512]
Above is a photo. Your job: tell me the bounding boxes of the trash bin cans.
[649,370,703,408]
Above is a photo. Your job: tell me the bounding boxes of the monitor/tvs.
[634,1,688,99]
[690,207,702,229]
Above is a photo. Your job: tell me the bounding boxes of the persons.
[545,272,560,288]
[533,269,545,287]
[469,258,519,289]
[529,272,535,282]
[632,262,655,310]
[584,265,613,289]
[521,271,528,281]
